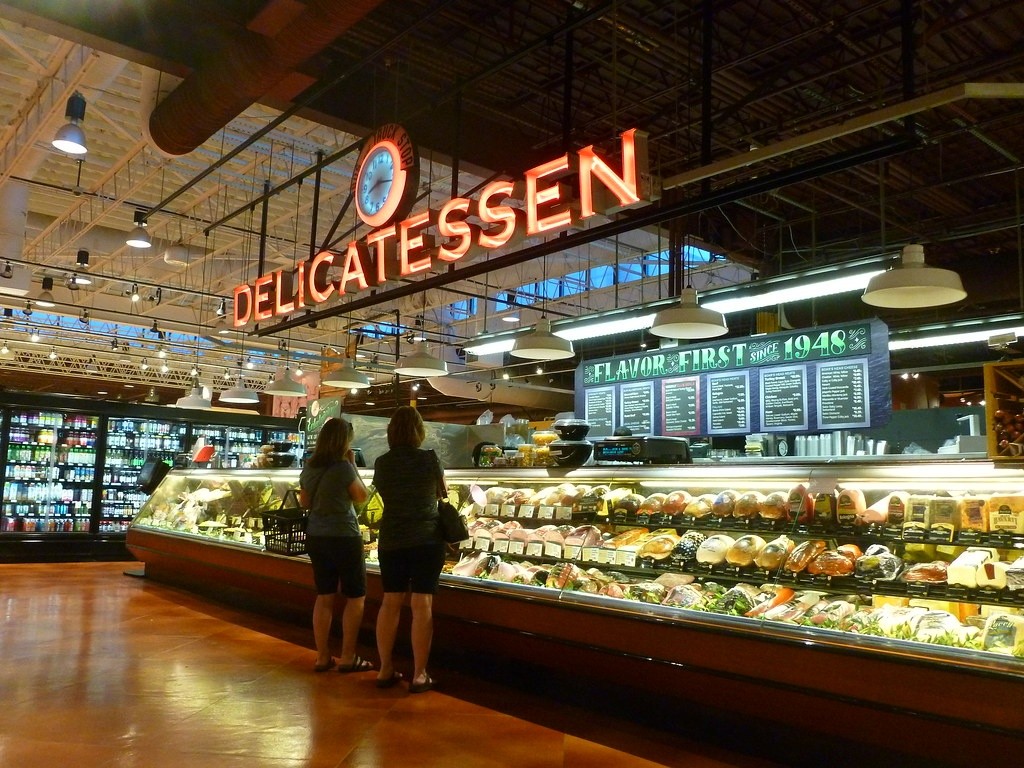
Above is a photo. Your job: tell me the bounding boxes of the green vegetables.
[623,578,983,652]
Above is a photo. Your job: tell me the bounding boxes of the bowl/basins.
[706,449,736,462]
[263,451,297,467]
[550,418,594,467]
[531,430,559,467]
[270,439,293,452]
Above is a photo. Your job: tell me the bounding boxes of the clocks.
[352,123,420,228]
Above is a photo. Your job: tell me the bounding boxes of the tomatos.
[994,409,1024,450]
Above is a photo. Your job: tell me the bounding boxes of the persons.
[299,418,376,672]
[372,406,448,693]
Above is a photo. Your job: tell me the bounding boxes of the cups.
[493,443,535,467]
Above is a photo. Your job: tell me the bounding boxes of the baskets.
[259,490,307,556]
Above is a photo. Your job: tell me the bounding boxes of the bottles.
[192,425,262,468]
[99,420,186,534]
[267,432,304,444]
[1,411,99,533]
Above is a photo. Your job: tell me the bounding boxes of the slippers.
[314,657,336,671]
[339,653,374,672]
[376,669,404,688]
[409,673,441,693]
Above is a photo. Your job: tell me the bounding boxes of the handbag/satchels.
[299,516,309,532]
[428,449,469,543]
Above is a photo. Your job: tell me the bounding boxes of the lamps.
[463,250,902,356]
[36,277,56,307]
[406,329,415,343]
[23,300,33,315]
[510,256,575,359]
[52,96,88,153]
[861,243,967,308]
[217,298,226,315]
[394,158,449,377]
[218,333,260,403]
[80,308,89,323]
[126,212,152,249]
[264,328,307,396]
[126,283,140,302]
[322,310,371,388]
[176,236,213,409]
[0,261,14,279]
[111,338,118,351]
[650,238,731,339]
[67,251,92,291]
[150,320,160,333]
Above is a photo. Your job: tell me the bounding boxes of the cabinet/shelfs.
[126,466,1024,768]
